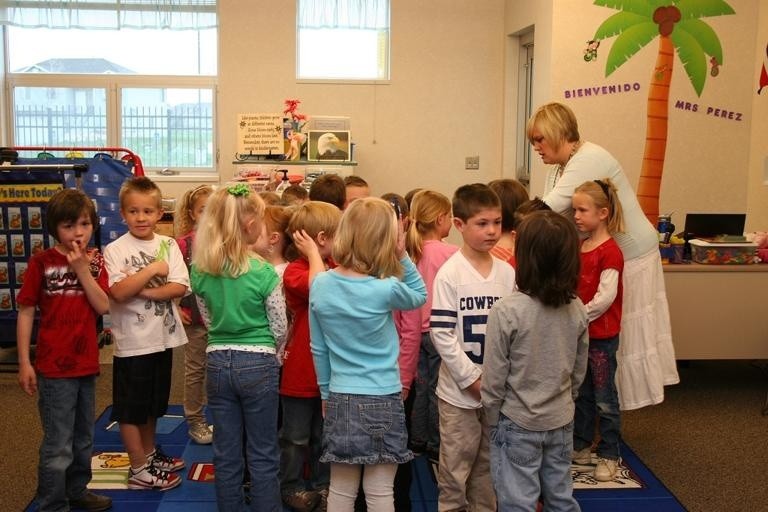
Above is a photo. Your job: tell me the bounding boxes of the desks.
[654,260,766,369]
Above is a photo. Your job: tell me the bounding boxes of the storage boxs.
[689,235,758,265]
[159,193,177,212]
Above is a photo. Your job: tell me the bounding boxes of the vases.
[290,122,300,160]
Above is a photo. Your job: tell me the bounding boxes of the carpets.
[22,400,690,510]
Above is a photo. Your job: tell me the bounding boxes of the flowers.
[283,98,307,131]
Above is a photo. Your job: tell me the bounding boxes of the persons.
[101,177,190,492]
[14,187,112,511]
[175,170,627,512]
[527,101,681,412]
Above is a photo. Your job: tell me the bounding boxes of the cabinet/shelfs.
[153,210,176,238]
[230,158,357,195]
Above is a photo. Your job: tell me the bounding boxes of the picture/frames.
[306,129,352,163]
[308,115,350,131]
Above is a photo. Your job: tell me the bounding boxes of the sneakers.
[147,451,185,473]
[70,490,111,511]
[573,442,623,482]
[127,465,181,492]
[188,421,213,445]
[281,488,329,512]
